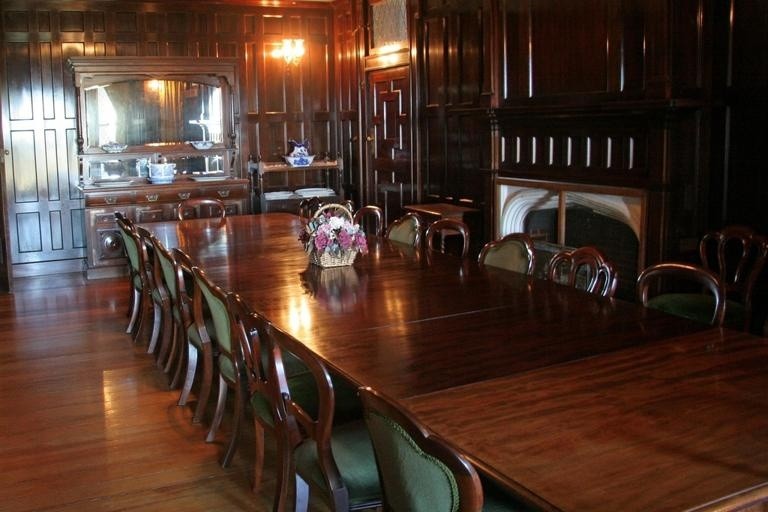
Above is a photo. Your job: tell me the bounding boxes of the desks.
[109,209,768,511]
[404,202,481,233]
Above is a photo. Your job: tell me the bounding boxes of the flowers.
[300,217,368,255]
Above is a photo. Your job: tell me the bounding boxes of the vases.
[309,252,358,267]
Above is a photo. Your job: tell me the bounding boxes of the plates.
[189,140,215,150]
[187,172,232,183]
[100,144,129,153]
[92,178,135,189]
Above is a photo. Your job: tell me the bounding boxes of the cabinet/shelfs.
[196,183,249,217]
[81,192,130,269]
[248,152,346,213]
[162,189,201,218]
[131,190,171,222]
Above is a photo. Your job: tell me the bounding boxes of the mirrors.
[75,72,239,153]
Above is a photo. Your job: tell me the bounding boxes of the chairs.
[477,233,537,277]
[148,235,177,366]
[178,196,227,219]
[334,200,354,219]
[636,264,725,328]
[385,212,424,248]
[424,218,470,261]
[353,205,383,236]
[173,248,210,423]
[191,264,242,470]
[357,383,482,512]
[549,247,618,298]
[263,323,381,510]
[696,225,768,333]
[134,227,157,354]
[226,292,289,511]
[113,212,146,339]
[299,196,325,218]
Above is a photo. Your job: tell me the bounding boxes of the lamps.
[274,37,307,62]
